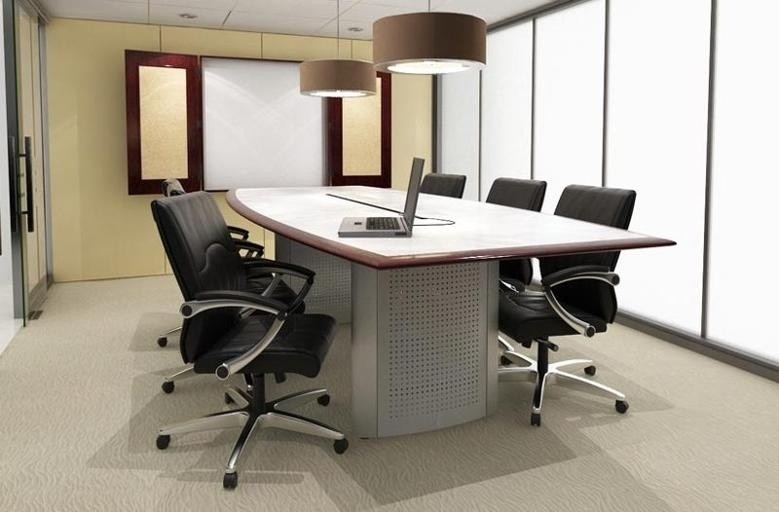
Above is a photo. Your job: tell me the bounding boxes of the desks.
[225,184,678,438]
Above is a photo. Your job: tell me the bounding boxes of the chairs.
[484,178,548,366]
[150,178,347,489]
[420,173,466,199]
[495,184,637,428]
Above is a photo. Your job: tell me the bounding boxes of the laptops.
[338,157,425,237]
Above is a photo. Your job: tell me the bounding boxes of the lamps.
[372,0,487,74]
[300,1,376,100]
